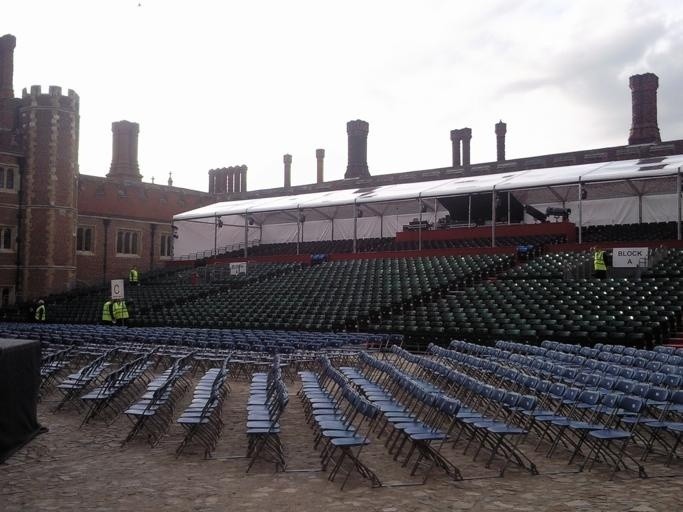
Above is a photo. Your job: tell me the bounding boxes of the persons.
[101,296,116,325]
[128,264,138,285]
[591,244,609,278]
[112,298,130,326]
[34,299,46,324]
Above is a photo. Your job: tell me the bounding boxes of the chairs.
[0,219,682,490]
